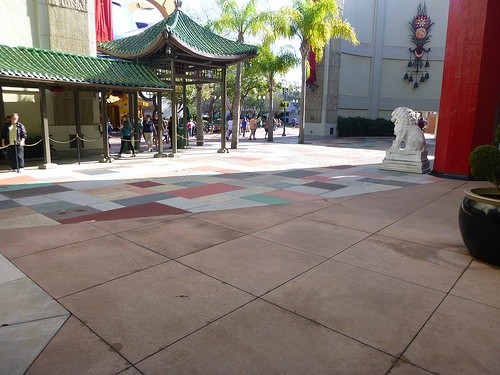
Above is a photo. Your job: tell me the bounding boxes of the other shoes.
[149,148,153,152]
[116,154,121,157]
[11,168,17,171]
[19,168,24,172]
[130,154,136,157]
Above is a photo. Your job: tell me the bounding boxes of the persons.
[417,117,425,131]
[137,118,143,144]
[265,117,269,139]
[258,116,263,128]
[186,118,197,138]
[141,115,157,152]
[249,115,257,140]
[239,121,243,134]
[1,112,28,173]
[273,118,284,131]
[242,116,246,137]
[162,116,172,149]
[152,110,159,146]
[107,117,113,136]
[225,118,233,140]
[114,115,136,159]
[246,118,250,132]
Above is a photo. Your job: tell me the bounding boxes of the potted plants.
[458,124,500,267]
[25,135,52,158]
